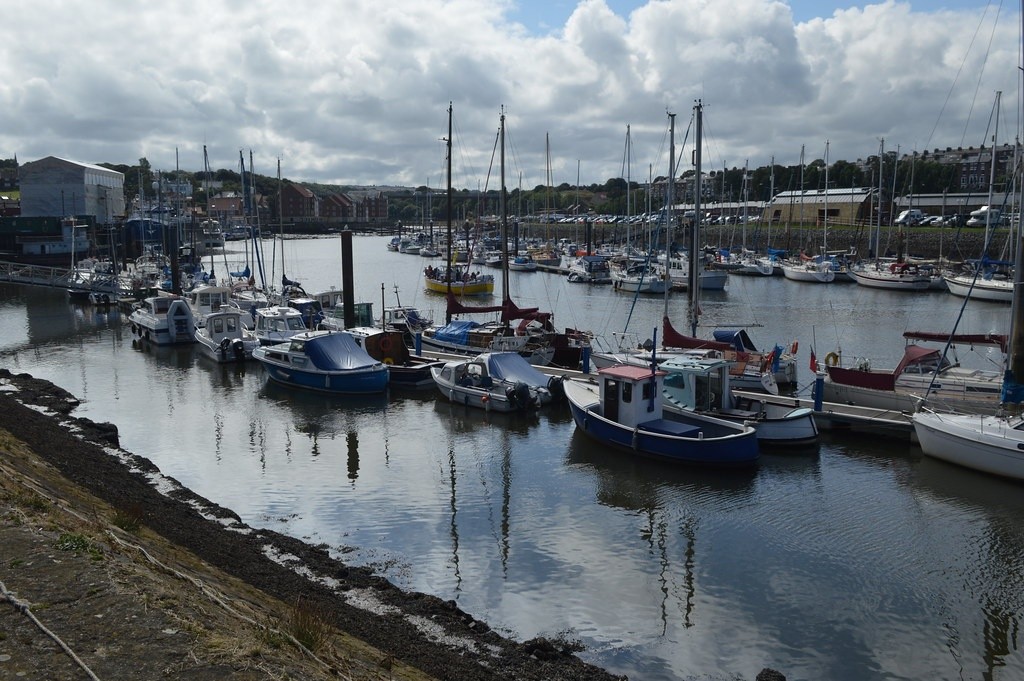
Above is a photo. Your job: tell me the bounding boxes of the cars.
[482,212,759,224]
[894,206,1024,227]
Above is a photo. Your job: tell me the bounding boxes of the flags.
[810,348,817,372]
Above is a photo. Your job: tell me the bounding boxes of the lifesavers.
[825,352,838,366]
[380,337,393,353]
[382,358,394,364]
[791,341,799,354]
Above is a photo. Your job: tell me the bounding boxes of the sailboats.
[783,141,836,280]
[707,160,773,276]
[849,140,931,291]
[943,90,1015,303]
[389,125,662,277]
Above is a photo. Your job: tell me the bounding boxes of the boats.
[658,264,729,289]
[604,166,675,292]
[569,254,612,283]
[65,155,996,480]
[508,256,537,270]
